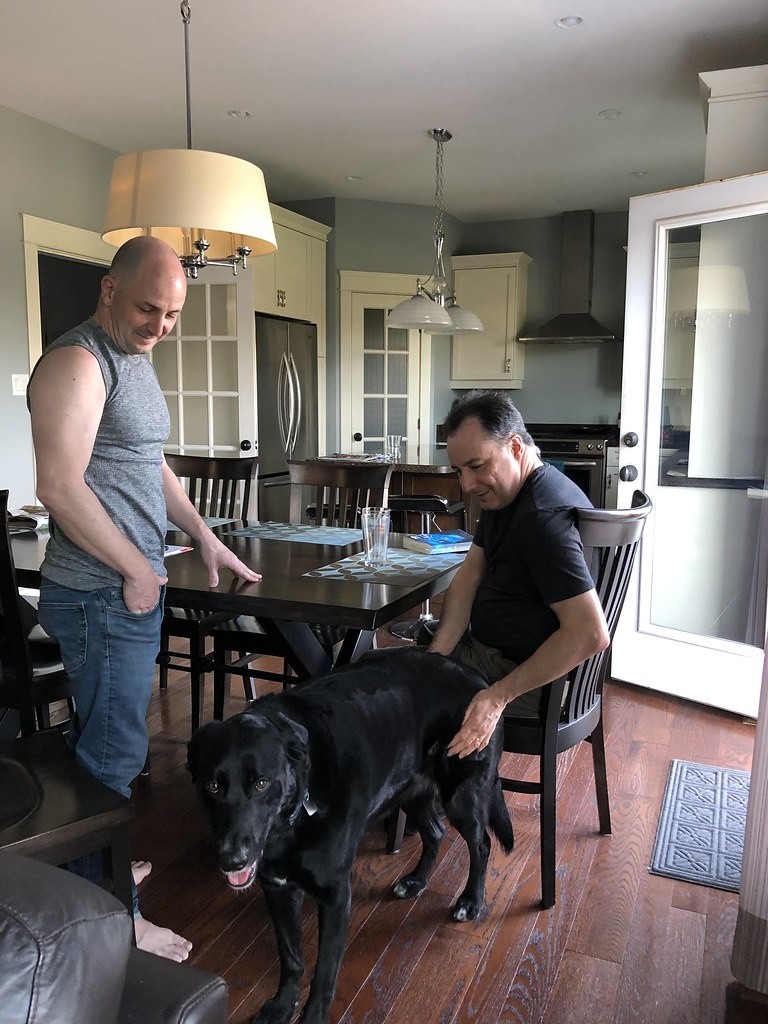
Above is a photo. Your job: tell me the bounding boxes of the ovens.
[531,437,608,509]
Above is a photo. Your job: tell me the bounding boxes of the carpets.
[648,758,753,893]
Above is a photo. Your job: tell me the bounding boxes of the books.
[402,528,474,555]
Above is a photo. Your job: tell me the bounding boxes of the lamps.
[100,0,278,278]
[387,128,485,335]
[668,265,749,338]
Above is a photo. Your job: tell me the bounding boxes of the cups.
[361,513,391,566]
[392,435,401,447]
[388,435,392,446]
[362,507,391,516]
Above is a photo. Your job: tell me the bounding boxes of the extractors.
[518,209,623,344]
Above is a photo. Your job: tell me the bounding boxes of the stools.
[305,503,362,528]
[387,493,465,641]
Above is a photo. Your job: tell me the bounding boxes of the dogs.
[186,645,515,1024]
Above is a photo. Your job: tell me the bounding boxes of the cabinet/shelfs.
[602,447,679,563]
[449,251,534,390]
[622,242,701,390]
[248,201,332,325]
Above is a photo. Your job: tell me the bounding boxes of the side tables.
[0,735,137,947]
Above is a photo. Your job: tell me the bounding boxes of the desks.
[10,515,471,684]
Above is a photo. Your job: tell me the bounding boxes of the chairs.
[159,453,396,737]
[1,489,75,740]
[386,490,653,909]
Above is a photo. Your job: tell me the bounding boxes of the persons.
[383,389,610,836]
[26,235,263,962]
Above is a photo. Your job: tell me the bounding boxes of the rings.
[476,738,483,743]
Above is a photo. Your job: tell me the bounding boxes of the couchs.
[0,849,230,1023]
